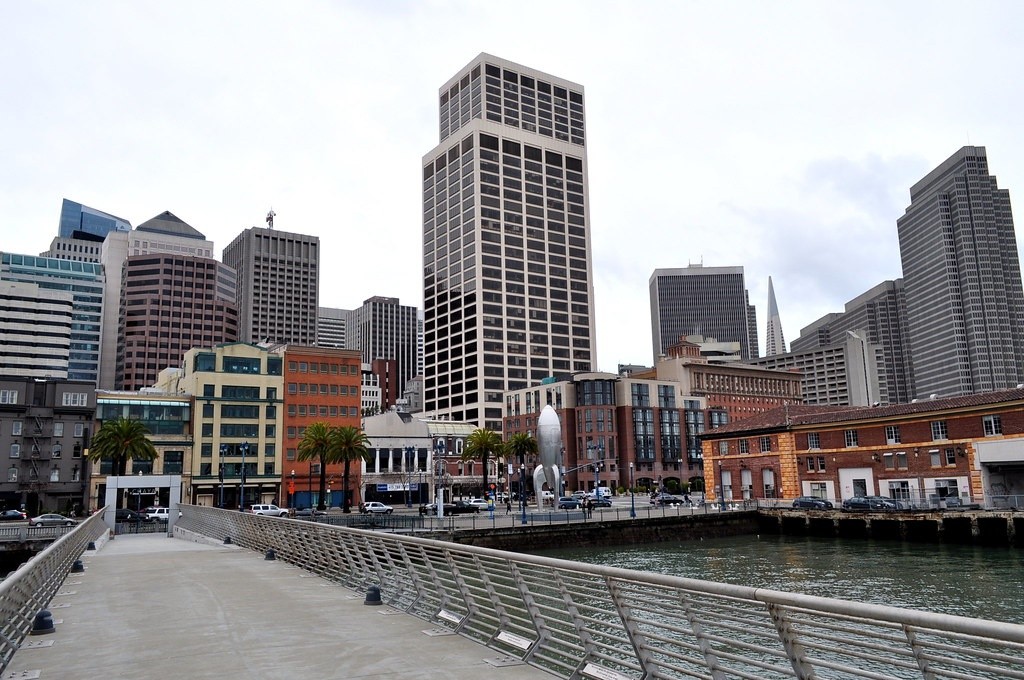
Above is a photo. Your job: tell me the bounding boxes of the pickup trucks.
[295,509,327,517]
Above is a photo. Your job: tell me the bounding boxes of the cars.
[498,492,516,501]
[650,493,684,506]
[0,510,27,520]
[29,514,78,527]
[423,499,496,516]
[793,495,904,510]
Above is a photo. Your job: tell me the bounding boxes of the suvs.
[250,504,289,517]
[582,495,612,508]
[570,490,590,499]
[553,497,582,509]
[102,508,182,524]
[364,502,394,515]
[514,490,534,501]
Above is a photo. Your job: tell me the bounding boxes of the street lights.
[848,329,870,407]
[517,464,527,524]
[138,471,142,511]
[405,446,422,517]
[291,469,294,512]
[630,462,636,518]
[220,441,248,514]
[678,459,683,492]
[718,460,726,510]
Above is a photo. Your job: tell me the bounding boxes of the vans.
[541,491,554,501]
[590,487,612,498]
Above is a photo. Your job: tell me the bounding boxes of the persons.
[506,501,511,515]
[419,504,424,517]
[684,494,688,506]
[651,493,660,499]
[587,500,593,518]
[582,503,586,519]
